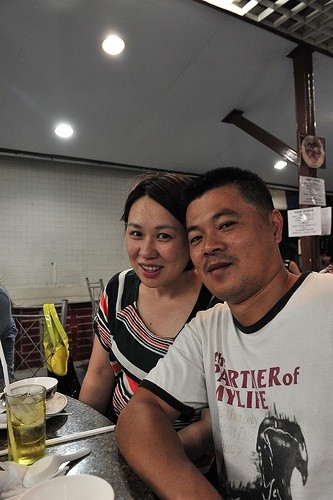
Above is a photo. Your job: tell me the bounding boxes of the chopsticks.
[0,425,116,455]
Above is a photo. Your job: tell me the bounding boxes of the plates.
[0,392,67,430]
[0,470,21,500]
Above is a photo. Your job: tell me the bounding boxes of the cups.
[5,384,46,466]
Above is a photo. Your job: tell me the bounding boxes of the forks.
[17,474,115,500]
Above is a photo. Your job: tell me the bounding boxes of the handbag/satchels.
[43,303,69,376]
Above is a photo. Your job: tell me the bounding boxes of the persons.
[283,248,333,275]
[0,285,18,392]
[78,173,220,492]
[113,167,333,500]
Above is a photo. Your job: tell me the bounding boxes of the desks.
[0,390,157,500]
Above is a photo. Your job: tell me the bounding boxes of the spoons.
[22,447,90,487]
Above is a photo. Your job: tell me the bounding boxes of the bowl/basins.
[3,377,58,409]
